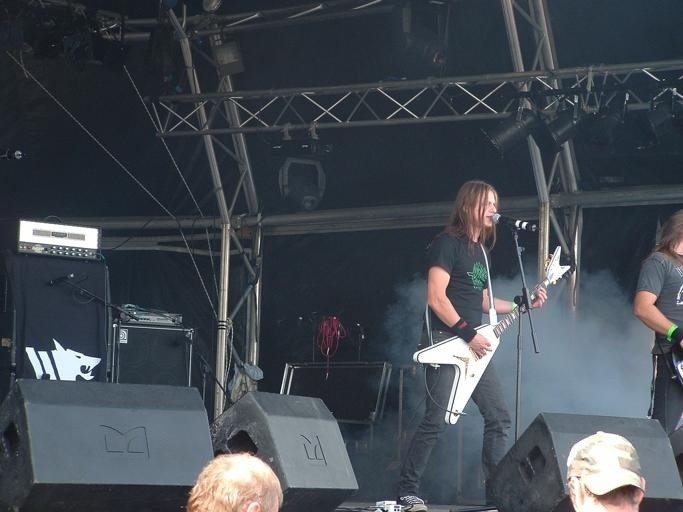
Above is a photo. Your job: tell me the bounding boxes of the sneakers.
[397,495,429,512]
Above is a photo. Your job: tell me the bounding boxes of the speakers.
[1,376,215,512]
[208,390,359,511]
[280,360,392,427]
[8,250,112,383]
[113,324,193,386]
[488,413,682,512]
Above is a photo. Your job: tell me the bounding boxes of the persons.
[567,431,646,512]
[397,180,548,512]
[634,208,683,485]
[185,452,283,512]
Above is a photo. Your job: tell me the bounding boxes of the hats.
[567,431,645,496]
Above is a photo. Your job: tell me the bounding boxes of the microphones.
[493,213,538,233]
[49,273,74,285]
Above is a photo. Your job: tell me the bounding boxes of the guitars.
[411,245,571,426]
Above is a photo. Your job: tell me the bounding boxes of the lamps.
[478,75,683,174]
[268,130,335,214]
[13,1,244,80]
[368,26,448,72]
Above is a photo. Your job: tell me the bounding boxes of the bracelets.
[512,301,517,311]
[666,324,678,342]
[671,327,683,343]
[450,317,477,343]
